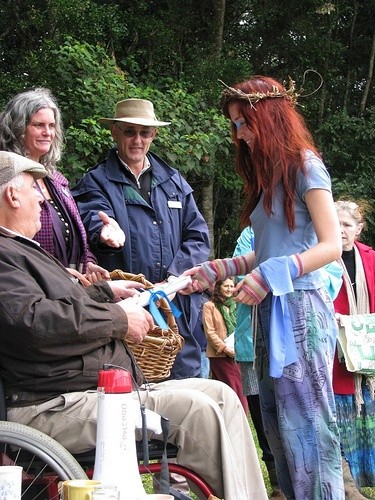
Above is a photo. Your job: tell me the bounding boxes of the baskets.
[80,269,185,382]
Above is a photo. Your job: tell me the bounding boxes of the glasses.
[116,125,153,138]
[336,201,360,214]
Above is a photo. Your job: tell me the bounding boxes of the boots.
[343,459,369,500]
[270,469,287,500]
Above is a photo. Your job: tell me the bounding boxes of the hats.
[0,151,47,187]
[99,99,171,126]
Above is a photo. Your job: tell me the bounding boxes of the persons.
[332,201,375,500]
[70,99,212,381]
[178,77,345,500]
[202,276,248,418]
[233,225,287,500]
[0,152,267,500]
[201,282,217,379]
[0,88,112,285]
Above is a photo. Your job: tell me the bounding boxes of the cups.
[62,480,103,500]
[0,466,23,500]
